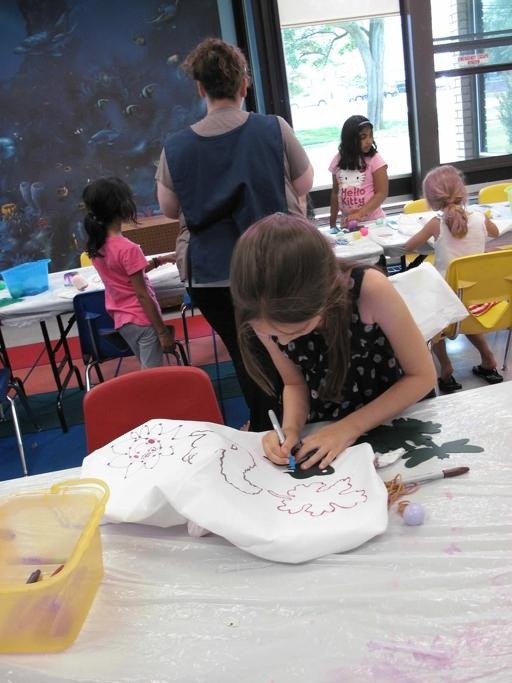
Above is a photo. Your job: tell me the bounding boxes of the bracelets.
[158,256,165,265]
[156,329,169,337]
[153,257,160,268]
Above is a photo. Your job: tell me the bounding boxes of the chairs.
[1,369,39,474]
[180,290,196,366]
[478,184,512,203]
[84,367,224,455]
[404,199,437,265]
[443,248,512,371]
[80,252,93,267]
[73,288,189,391]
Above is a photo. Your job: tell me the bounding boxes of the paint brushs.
[27,567,42,585]
[266,407,296,474]
[399,465,466,485]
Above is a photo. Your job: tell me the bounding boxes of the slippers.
[437,375,462,391]
[472,364,503,384]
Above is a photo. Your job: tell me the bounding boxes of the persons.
[155,37,315,432]
[403,164,501,383]
[325,115,390,232]
[80,176,176,371]
[228,210,438,471]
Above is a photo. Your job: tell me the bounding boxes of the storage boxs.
[0,259,51,299]
[0,477,111,655]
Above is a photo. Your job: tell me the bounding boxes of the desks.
[1,224,383,433]
[0,379,511,682]
[357,201,512,270]
